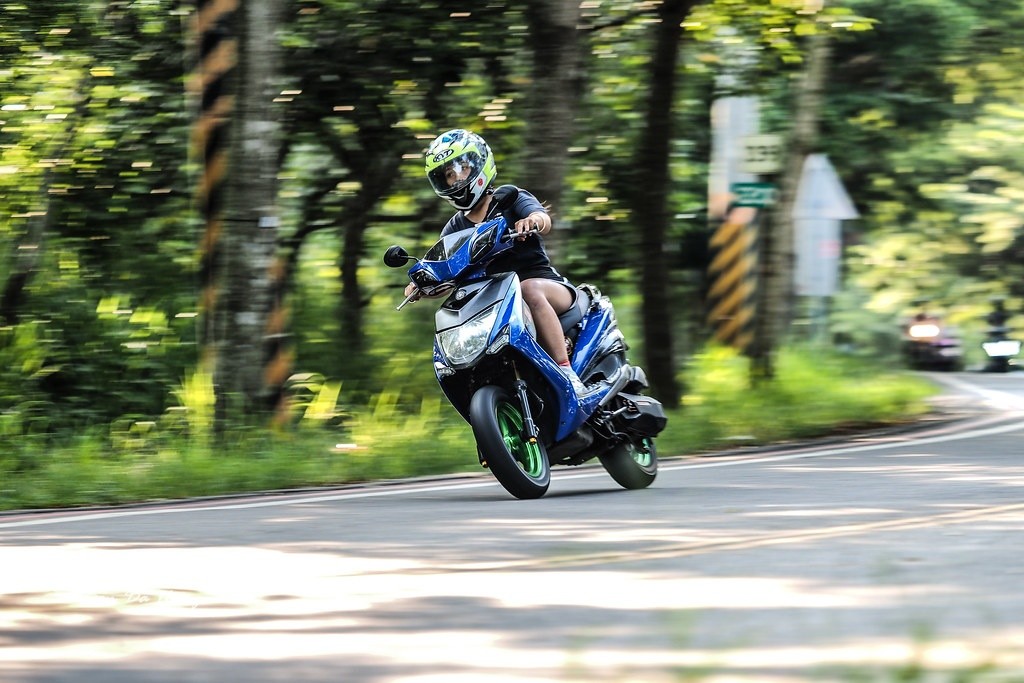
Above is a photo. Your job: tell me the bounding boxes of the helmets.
[424,128,498,212]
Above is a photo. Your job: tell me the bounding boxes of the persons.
[405,129,590,396]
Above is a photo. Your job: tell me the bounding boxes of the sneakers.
[559,366,589,395]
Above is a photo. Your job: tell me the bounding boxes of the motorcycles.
[384,185,668,499]
